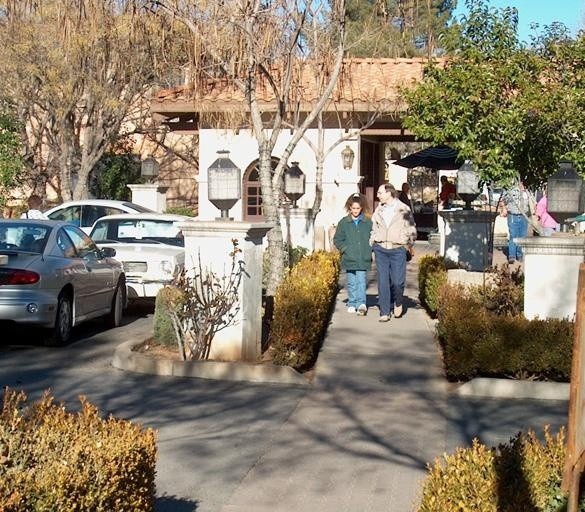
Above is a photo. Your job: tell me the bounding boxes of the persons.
[398,183,416,256]
[440,176,456,208]
[333,194,371,315]
[369,184,418,322]
[499,181,537,263]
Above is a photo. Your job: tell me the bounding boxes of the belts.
[508,212,524,216]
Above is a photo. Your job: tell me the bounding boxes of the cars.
[0,200,193,347]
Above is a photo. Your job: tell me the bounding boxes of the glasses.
[378,191,385,194]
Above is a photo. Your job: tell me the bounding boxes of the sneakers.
[508,256,522,263]
[379,301,403,322]
[347,304,367,316]
